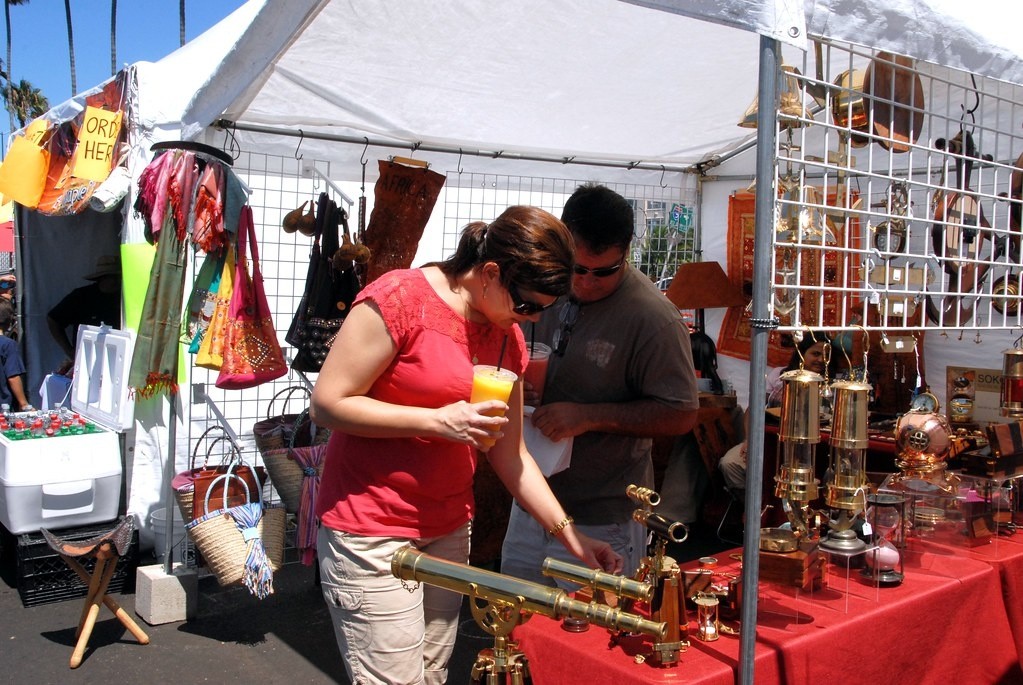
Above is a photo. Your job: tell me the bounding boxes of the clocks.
[908,385,942,414]
[873,223,906,260]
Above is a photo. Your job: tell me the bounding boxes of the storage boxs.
[961,419,1023,479]
[0,324,139,608]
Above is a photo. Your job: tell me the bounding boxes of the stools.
[38,516,150,669]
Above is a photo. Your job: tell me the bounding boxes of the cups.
[524,341,552,408]
[469,364,518,448]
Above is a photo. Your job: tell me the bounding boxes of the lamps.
[663,263,744,379]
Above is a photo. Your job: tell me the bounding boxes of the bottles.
[0,403,102,441]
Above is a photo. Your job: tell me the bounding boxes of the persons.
[310,206,624,685]
[718,332,838,520]
[0,270,37,418]
[499,186,699,636]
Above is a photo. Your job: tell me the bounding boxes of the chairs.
[691,387,747,544]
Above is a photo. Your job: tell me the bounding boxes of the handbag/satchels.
[192,443,268,521]
[253,386,312,453]
[284,191,330,350]
[184,459,286,588]
[290,208,362,373]
[216,204,288,390]
[189,229,237,353]
[171,426,242,525]
[262,407,330,514]
[195,233,252,372]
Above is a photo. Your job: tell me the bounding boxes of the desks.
[504,513,1023,685]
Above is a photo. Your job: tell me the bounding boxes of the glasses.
[571,252,625,278]
[509,288,559,316]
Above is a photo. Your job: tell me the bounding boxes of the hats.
[0,275,16,282]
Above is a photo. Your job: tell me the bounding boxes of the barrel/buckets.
[149,506,195,566]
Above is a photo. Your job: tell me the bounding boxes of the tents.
[90,0,1023,574]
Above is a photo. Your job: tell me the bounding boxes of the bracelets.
[548,516,574,536]
